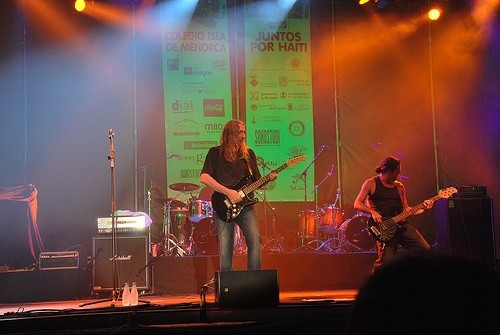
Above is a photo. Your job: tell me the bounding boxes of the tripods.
[292,145,366,253]
[255,191,286,254]
[79,135,151,308]
[156,188,188,256]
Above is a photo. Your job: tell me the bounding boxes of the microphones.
[109,129,114,137]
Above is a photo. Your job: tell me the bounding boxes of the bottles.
[130,282,138,306]
[122,283,130,306]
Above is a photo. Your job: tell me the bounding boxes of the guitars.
[367,186,458,243]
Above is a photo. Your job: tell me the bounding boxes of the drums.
[160,208,193,238]
[316,206,344,233]
[191,216,239,255]
[298,209,320,240]
[189,199,210,223]
[337,213,376,252]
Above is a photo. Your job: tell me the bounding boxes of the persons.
[185,187,220,294]
[200,120,277,272]
[353,155,434,271]
[351,255,482,335]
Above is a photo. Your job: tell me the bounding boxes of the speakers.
[215,269,279,310]
[434,197,496,269]
[92,235,150,290]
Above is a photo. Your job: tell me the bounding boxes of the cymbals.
[153,197,188,207]
[144,175,164,196]
[254,187,268,191]
[169,182,200,193]
[396,175,411,180]
